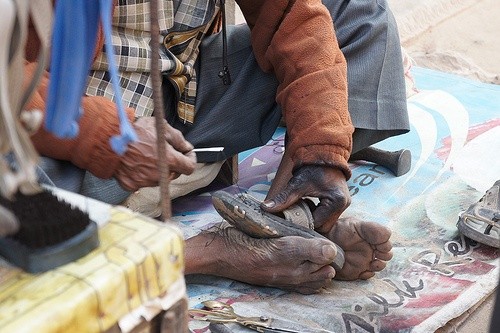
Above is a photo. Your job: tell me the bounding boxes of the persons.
[3,0,412,296]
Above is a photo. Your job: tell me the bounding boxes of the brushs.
[0,188,101,275]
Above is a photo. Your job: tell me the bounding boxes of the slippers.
[457,180,500,251]
[210,187,347,273]
